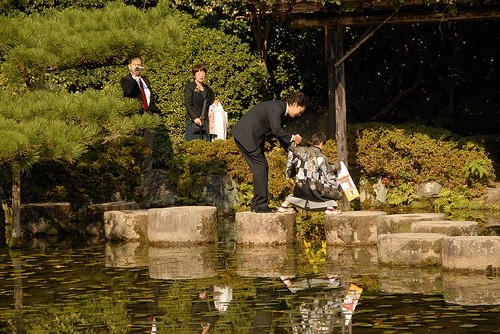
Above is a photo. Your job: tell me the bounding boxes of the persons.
[120,54,153,113]
[284,131,349,215]
[231,92,308,215]
[184,64,218,143]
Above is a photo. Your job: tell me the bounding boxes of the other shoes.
[251,207,257,212]
[256,207,276,213]
[325,208,342,215]
[278,205,294,212]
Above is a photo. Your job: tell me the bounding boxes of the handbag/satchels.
[192,117,207,135]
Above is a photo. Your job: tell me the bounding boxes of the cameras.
[134,66,145,71]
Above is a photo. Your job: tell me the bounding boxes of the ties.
[139,78,149,110]
